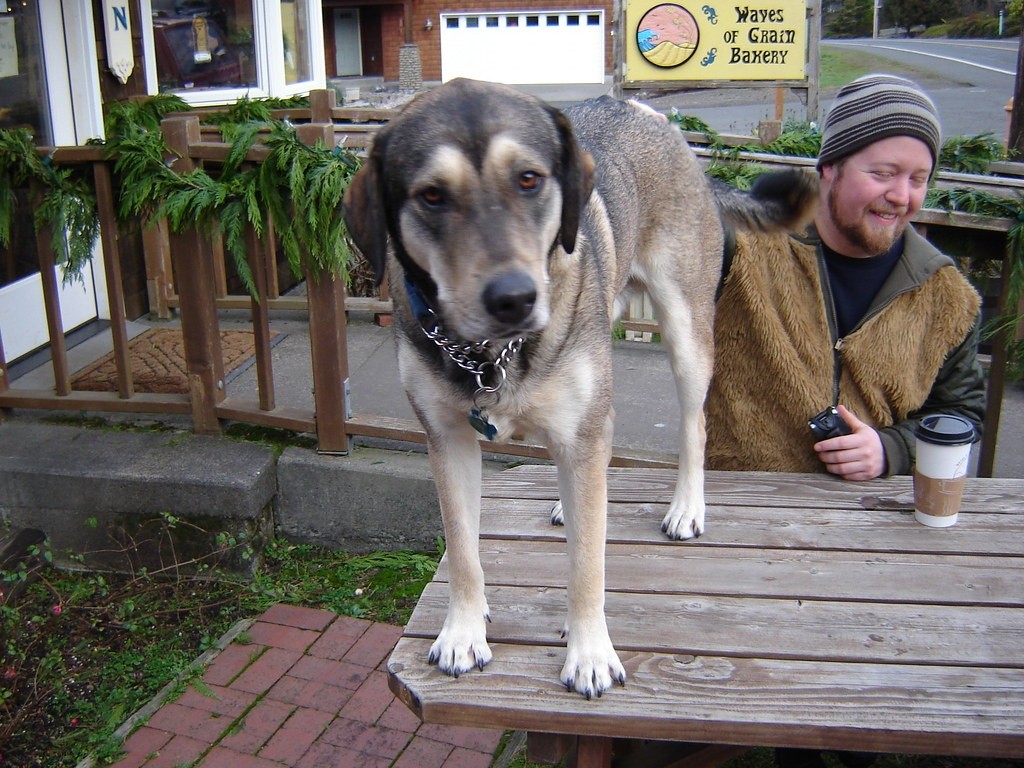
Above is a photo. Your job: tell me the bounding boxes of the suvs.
[98,10,243,107]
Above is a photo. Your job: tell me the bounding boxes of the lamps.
[424,16,433,32]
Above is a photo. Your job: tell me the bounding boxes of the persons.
[698,74,988,768]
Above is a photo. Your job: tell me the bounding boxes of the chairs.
[910,210,1017,477]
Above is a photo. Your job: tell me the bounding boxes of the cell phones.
[808,405,851,442]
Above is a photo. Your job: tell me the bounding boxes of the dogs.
[335,75,727,703]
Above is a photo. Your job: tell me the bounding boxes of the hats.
[816,73,940,182]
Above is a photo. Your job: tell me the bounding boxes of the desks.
[385,459,1024,768]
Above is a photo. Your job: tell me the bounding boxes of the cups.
[911,414,976,527]
